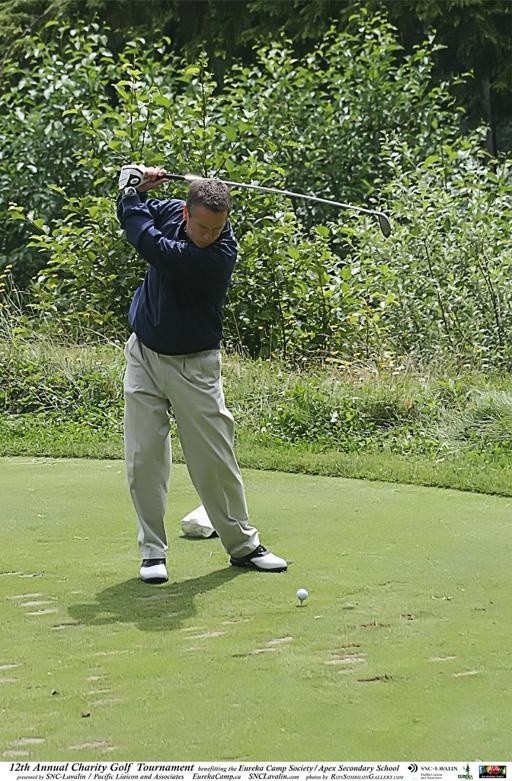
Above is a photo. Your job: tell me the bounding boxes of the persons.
[117,165,288,582]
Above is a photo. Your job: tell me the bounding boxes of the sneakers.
[140,559,168,583]
[230,545,288,572]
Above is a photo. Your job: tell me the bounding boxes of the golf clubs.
[164,174,392,239]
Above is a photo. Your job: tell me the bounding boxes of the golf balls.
[297,590,307,601]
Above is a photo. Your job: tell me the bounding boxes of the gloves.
[118,164,147,191]
